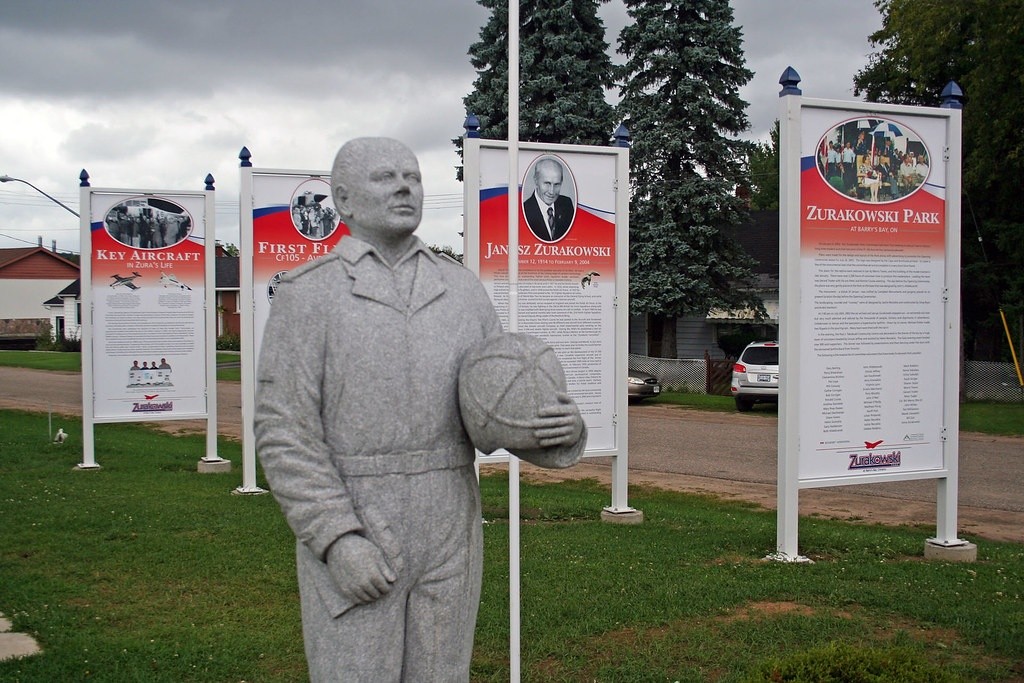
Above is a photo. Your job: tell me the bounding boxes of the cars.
[731,339,779,412]
[628,369,663,403]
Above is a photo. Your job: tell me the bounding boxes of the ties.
[547,207,555,241]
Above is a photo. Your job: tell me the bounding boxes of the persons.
[827,130,928,202]
[255,138,587,682]
[293,204,336,238]
[107,213,189,248]
[524,160,575,241]
